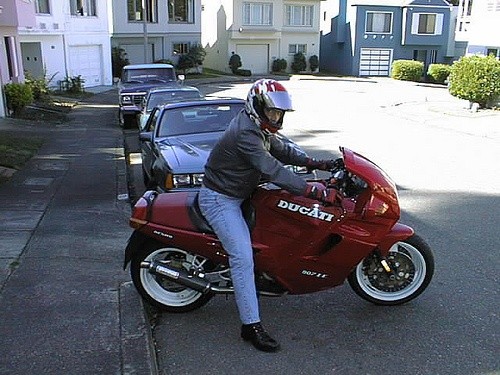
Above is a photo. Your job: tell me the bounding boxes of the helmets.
[245,78,295,135]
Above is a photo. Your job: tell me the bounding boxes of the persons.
[198,79,344,353]
[229,51,241,75]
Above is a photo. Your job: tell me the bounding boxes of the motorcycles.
[123,146,436,315]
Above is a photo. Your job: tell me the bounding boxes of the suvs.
[114,63,185,129]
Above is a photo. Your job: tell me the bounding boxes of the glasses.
[265,108,283,117]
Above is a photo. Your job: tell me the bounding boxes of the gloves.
[304,183,344,206]
[307,157,344,172]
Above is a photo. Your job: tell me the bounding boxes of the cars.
[138,96,246,195]
[136,85,214,132]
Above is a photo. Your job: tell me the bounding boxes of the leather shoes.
[241,323,280,351]
